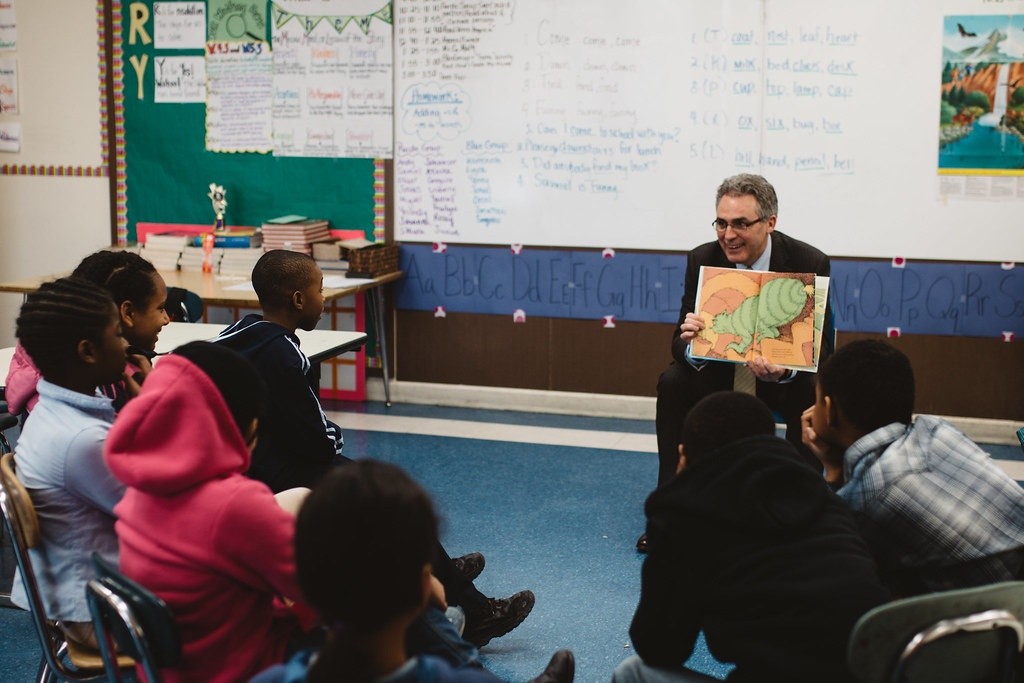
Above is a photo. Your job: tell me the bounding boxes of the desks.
[0,267,403,408]
[153,320,370,401]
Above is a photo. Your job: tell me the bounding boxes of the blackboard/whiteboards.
[390,0,1024,263]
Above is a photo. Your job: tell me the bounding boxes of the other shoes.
[528,649,575,683]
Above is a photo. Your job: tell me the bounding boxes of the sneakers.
[452,552,485,582]
[462,590,535,649]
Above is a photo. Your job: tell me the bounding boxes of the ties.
[733,267,757,397]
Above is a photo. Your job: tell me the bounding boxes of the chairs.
[843,581,1024,683]
[166,288,204,323]
[0,397,192,683]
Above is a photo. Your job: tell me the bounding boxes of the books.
[139,214,400,278]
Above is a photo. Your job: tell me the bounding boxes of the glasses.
[712,215,768,233]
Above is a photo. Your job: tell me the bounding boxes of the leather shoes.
[637,533,649,552]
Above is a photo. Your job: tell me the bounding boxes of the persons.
[803,339,1024,597]
[5,250,170,419]
[636,173,835,551]
[105,340,465,683]
[612,392,876,683]
[214,251,535,652]
[8,278,311,653]
[252,459,500,683]
